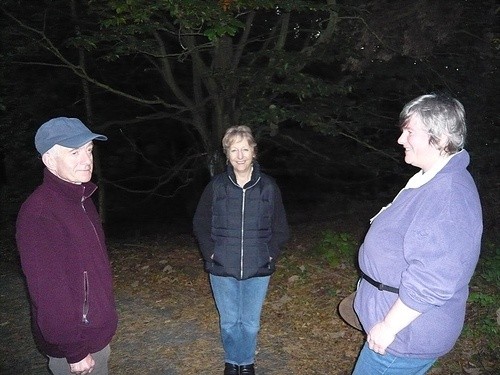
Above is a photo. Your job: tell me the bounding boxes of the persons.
[14,115,120,375]
[193,126,291,375]
[337,92,486,374]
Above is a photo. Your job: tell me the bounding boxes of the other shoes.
[224,362,240,375]
[240,362,256,375]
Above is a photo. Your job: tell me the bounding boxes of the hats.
[35,116,107,155]
